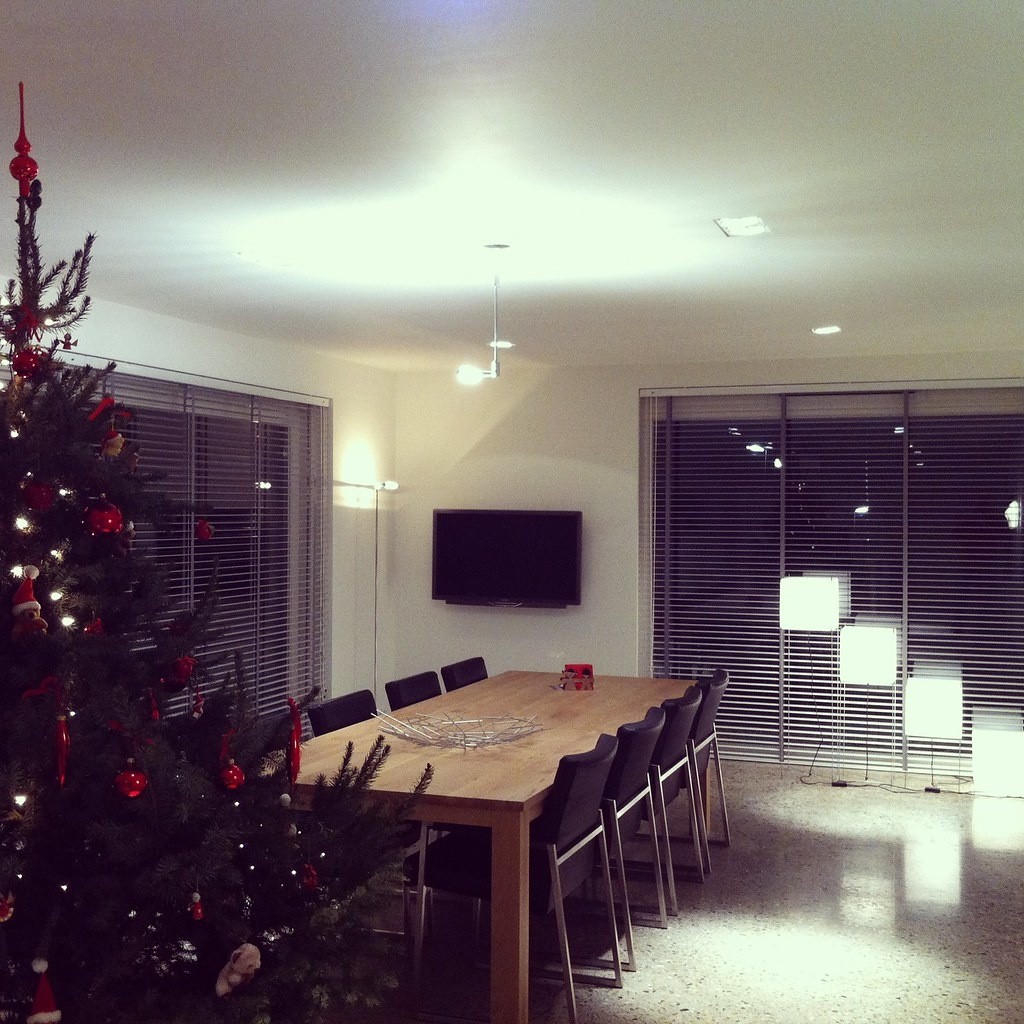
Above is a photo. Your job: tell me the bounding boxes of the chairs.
[570,682,705,918]
[402,735,623,1024]
[237,715,417,946]
[442,659,487,694]
[305,687,421,848]
[383,673,442,712]
[613,670,732,873]
[527,703,669,971]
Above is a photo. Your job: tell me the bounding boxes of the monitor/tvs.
[432,509,583,610]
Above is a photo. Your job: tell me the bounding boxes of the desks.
[259,672,714,1024]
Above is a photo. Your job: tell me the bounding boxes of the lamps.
[453,242,500,383]
